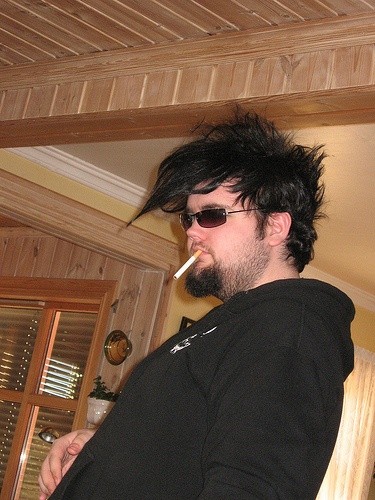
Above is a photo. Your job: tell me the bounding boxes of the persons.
[37,104,355,500]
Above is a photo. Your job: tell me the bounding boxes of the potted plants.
[86,375,118,426]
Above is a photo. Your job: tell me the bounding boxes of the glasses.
[178,207,261,232]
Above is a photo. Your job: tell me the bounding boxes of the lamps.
[38,428,58,445]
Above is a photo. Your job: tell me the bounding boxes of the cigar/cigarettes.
[173,250,203,281]
